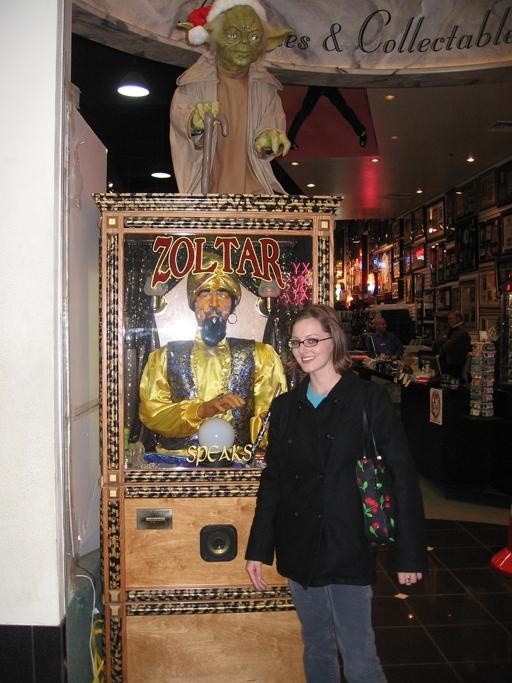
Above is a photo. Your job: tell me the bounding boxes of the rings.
[406,577,411,582]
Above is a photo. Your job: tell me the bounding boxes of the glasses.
[288,337,332,348]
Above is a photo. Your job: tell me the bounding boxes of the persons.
[139,261,288,455]
[437,309,472,379]
[288,85,367,153]
[366,316,403,359]
[170,0,294,195]
[245,306,428,678]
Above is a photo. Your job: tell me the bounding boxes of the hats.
[187,260,241,311]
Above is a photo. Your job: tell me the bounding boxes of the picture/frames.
[390,158,511,340]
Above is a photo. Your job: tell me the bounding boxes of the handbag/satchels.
[356,458,399,547]
